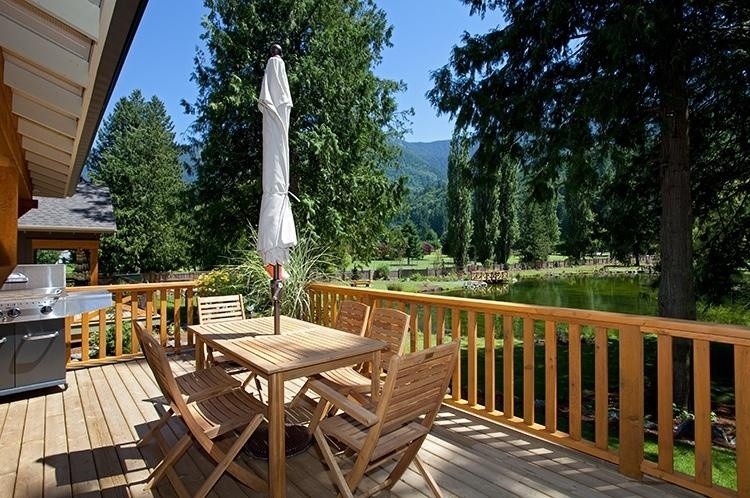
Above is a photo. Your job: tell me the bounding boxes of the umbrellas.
[255,43,299,336]
